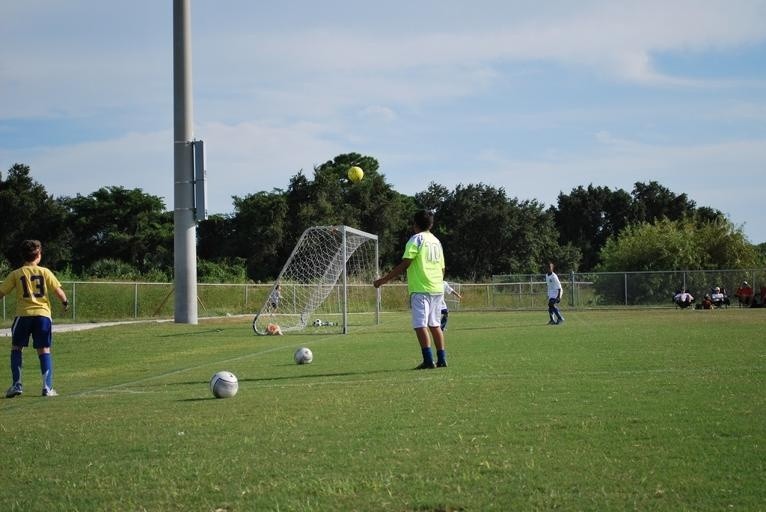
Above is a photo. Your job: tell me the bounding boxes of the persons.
[545,262,565,325]
[441,280,463,332]
[0,239,70,399]
[373,209,448,370]
[269,284,284,317]
[673,280,754,309]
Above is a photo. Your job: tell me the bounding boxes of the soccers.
[294,347,312,365]
[348,166,363,182]
[313,319,321,327]
[209,371,238,398]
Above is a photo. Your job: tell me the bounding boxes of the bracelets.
[61,299,68,306]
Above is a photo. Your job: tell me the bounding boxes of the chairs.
[671,285,766,311]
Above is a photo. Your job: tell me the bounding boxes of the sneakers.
[416,359,448,368]
[6,383,22,398]
[548,317,563,324]
[43,387,58,396]
